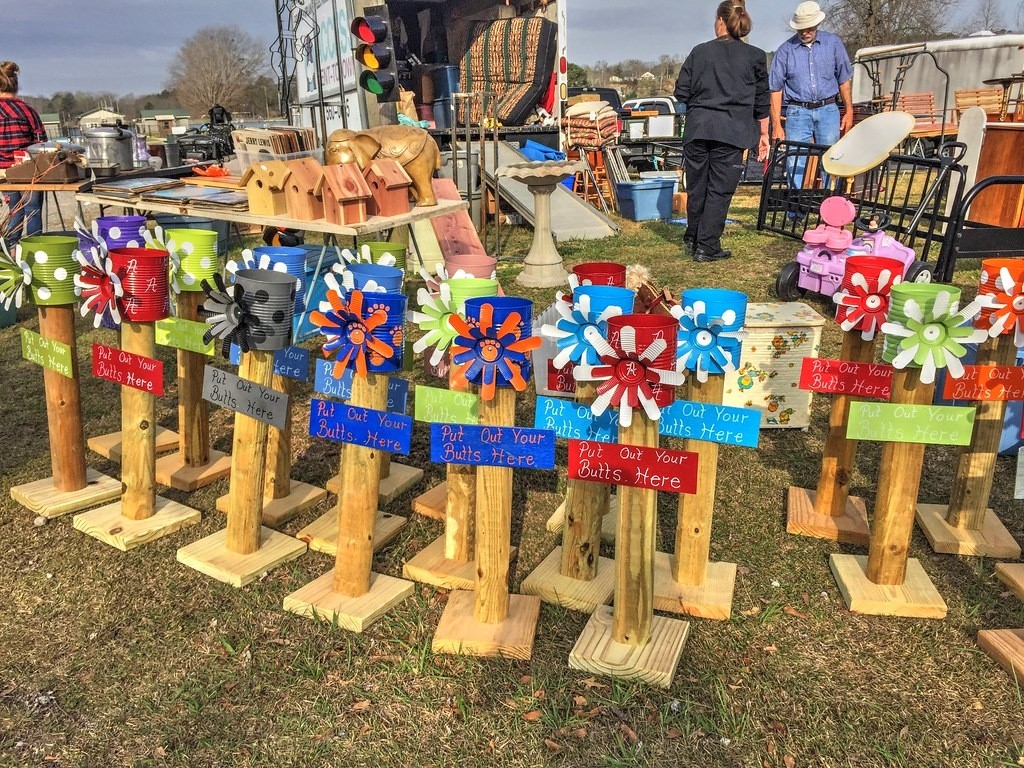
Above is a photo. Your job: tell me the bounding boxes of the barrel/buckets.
[148,141,180,168]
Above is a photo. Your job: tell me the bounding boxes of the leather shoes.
[685,242,700,255]
[693,248,731,262]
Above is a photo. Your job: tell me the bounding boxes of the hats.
[790,1,825,30]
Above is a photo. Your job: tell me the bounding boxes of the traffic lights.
[352,3,401,104]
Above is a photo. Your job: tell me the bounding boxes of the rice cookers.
[85,119,134,171]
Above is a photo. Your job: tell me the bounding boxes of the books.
[231,126,318,175]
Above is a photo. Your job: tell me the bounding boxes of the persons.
[766,2,853,225]
[672,0,771,263]
[0,61,48,256]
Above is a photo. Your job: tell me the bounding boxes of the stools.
[572,147,615,213]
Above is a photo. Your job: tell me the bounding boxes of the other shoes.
[788,216,802,227]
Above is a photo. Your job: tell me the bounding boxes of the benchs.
[879,88,1008,170]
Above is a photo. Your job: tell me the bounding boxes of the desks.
[0,158,473,346]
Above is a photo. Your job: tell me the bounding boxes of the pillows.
[562,100,619,150]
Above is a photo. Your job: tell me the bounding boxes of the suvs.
[568,86,621,112]
[621,96,681,115]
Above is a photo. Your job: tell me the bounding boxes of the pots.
[20,142,86,180]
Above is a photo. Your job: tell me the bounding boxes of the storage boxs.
[412,64,462,130]
[233,148,325,175]
[436,150,480,192]
[531,300,579,399]
[460,186,486,233]
[673,193,688,214]
[0,280,16,328]
[935,320,1024,458]
[640,170,681,193]
[615,177,676,222]
[153,212,227,257]
[644,114,675,138]
[488,187,514,215]
[291,244,357,341]
[498,212,526,225]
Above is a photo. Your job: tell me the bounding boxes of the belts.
[788,97,837,109]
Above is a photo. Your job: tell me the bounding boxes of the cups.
[572,263,626,289]
[606,314,679,409]
[234,270,297,351]
[107,247,170,322]
[357,241,407,296]
[164,229,219,292]
[343,292,410,372]
[438,278,499,320]
[971,258,1024,336]
[573,286,635,366]
[835,256,905,331]
[464,296,534,386]
[882,283,962,369]
[682,288,749,374]
[252,246,307,314]
[445,255,497,279]
[95,216,148,250]
[18,236,83,305]
[346,264,403,293]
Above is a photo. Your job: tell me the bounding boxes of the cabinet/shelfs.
[721,301,826,432]
